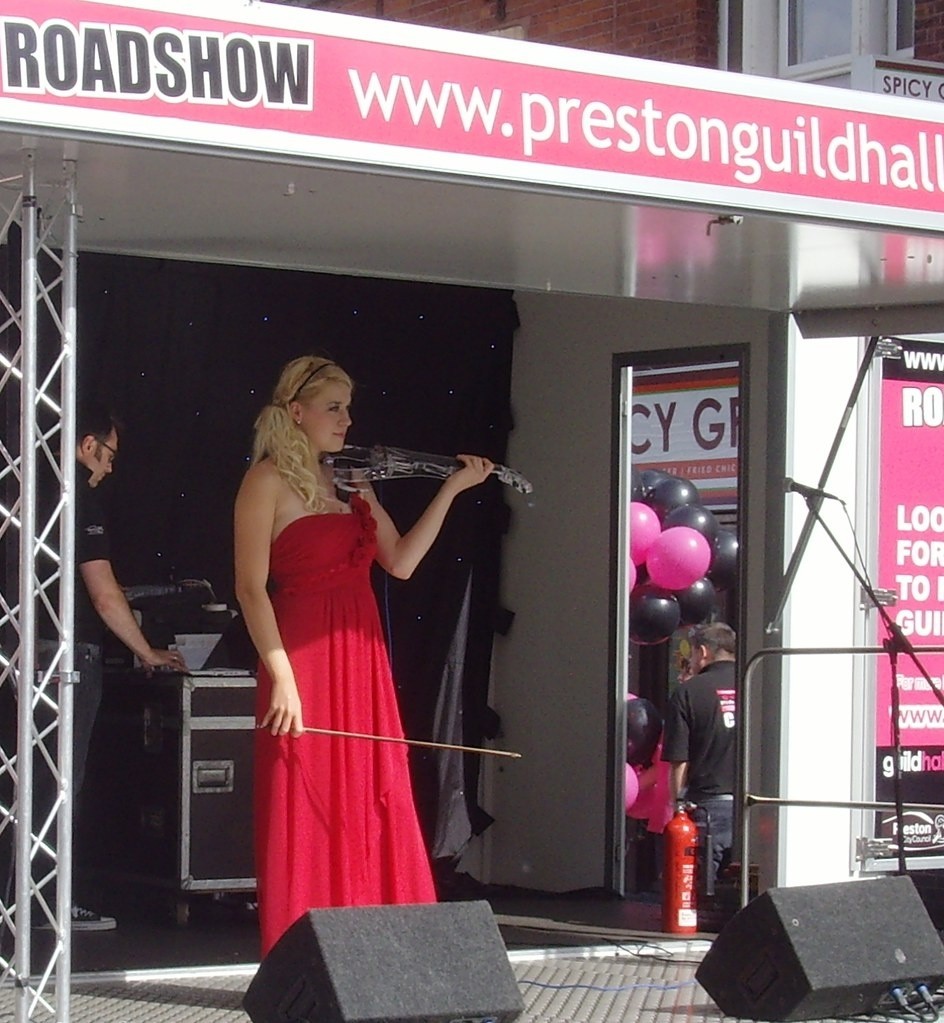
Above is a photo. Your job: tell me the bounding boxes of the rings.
[172,656,177,662]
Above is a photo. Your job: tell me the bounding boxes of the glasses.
[92,435,119,463]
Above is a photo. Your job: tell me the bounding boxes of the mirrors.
[608,338,753,903]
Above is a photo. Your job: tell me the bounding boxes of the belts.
[699,793,735,800]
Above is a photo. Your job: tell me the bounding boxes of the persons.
[667,620,736,892]
[21,407,187,932]
[234,355,495,962]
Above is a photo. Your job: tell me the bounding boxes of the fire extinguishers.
[663,797,716,936]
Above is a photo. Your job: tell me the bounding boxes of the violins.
[320,443,536,495]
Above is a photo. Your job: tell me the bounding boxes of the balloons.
[625,691,674,834]
[628,471,739,644]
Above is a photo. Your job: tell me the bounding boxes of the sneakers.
[31,902,116,931]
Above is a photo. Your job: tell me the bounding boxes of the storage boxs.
[79,669,259,929]
